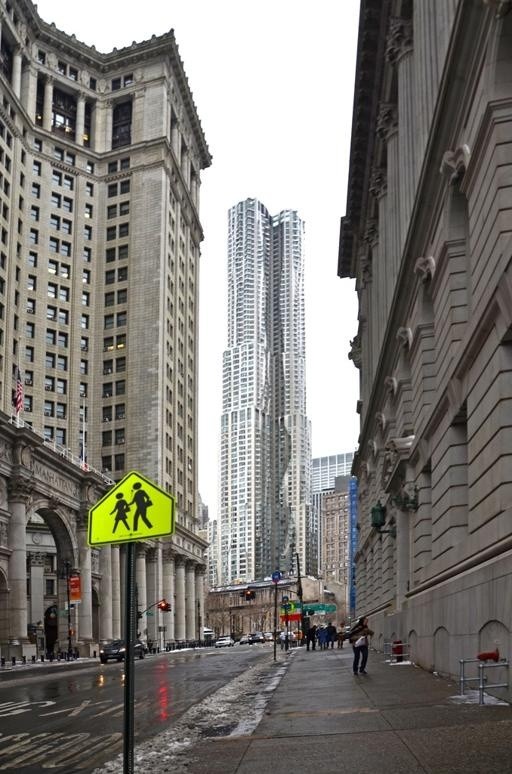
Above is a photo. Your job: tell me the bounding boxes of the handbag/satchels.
[355,636,366,648]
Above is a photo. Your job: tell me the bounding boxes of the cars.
[239,631,301,645]
[215,636,235,648]
[98,638,148,663]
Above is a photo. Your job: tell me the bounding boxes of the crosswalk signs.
[86,471,174,546]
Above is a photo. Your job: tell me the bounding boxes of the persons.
[350,617,368,677]
[306,623,345,651]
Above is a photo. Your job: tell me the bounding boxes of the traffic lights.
[238,588,256,601]
[157,600,172,613]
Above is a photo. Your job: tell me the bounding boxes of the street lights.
[57,559,80,661]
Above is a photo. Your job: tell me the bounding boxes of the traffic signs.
[292,603,301,608]
[303,603,325,611]
[325,604,336,611]
